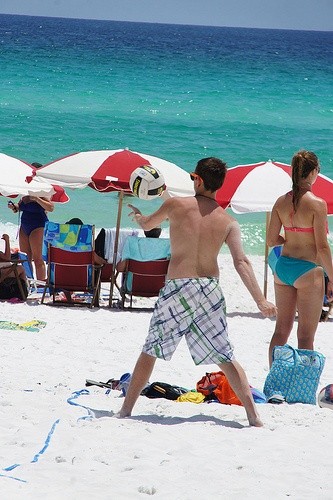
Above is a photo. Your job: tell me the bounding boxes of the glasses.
[317,167,320,174]
[189,172,204,183]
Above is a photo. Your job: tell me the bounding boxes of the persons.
[65,218,106,265]
[118,157,279,427]
[8,163,54,281]
[116,227,162,272]
[268,151,333,369]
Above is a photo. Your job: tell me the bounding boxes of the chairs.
[0,221,170,310]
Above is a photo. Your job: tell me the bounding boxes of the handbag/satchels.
[196,371,244,405]
[262,344,325,405]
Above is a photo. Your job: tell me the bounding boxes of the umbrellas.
[216,159,333,299]
[0,152,70,204]
[25,148,195,307]
